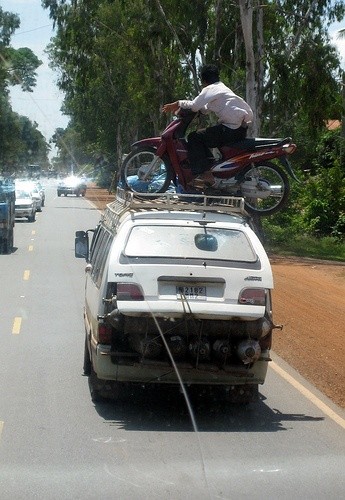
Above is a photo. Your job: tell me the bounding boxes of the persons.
[162,64,253,191]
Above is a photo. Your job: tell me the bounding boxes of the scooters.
[120,106,303,217]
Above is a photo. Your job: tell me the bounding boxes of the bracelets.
[176,100,179,107]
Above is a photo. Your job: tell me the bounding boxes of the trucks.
[0,172,17,254]
[27,165,40,180]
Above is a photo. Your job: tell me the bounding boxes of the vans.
[73,185,285,407]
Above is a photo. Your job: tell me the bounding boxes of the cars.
[123,165,177,199]
[14,182,45,222]
[57,177,87,197]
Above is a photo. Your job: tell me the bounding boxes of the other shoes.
[202,177,221,202]
